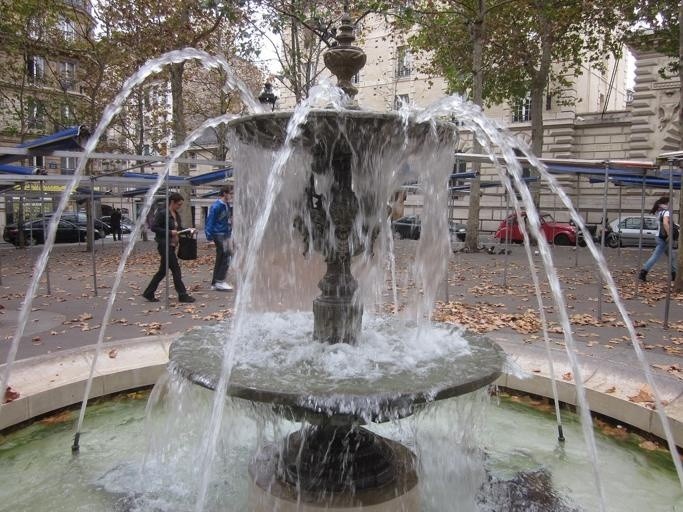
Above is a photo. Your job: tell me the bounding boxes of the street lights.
[257,79,278,111]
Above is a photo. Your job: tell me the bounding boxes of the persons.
[638,196,678,281]
[204,188,236,291]
[110,207,121,242]
[139,192,196,303]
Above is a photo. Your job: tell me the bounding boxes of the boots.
[672,272,676,281]
[639,270,648,281]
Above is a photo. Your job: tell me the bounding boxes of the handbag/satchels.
[177,234,197,260]
[659,210,680,240]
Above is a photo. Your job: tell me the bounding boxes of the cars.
[494,212,576,245]
[393,214,465,239]
[599,217,657,246]
[3,204,135,245]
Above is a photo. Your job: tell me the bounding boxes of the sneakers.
[179,294,196,302]
[211,282,233,290]
[143,288,159,302]
